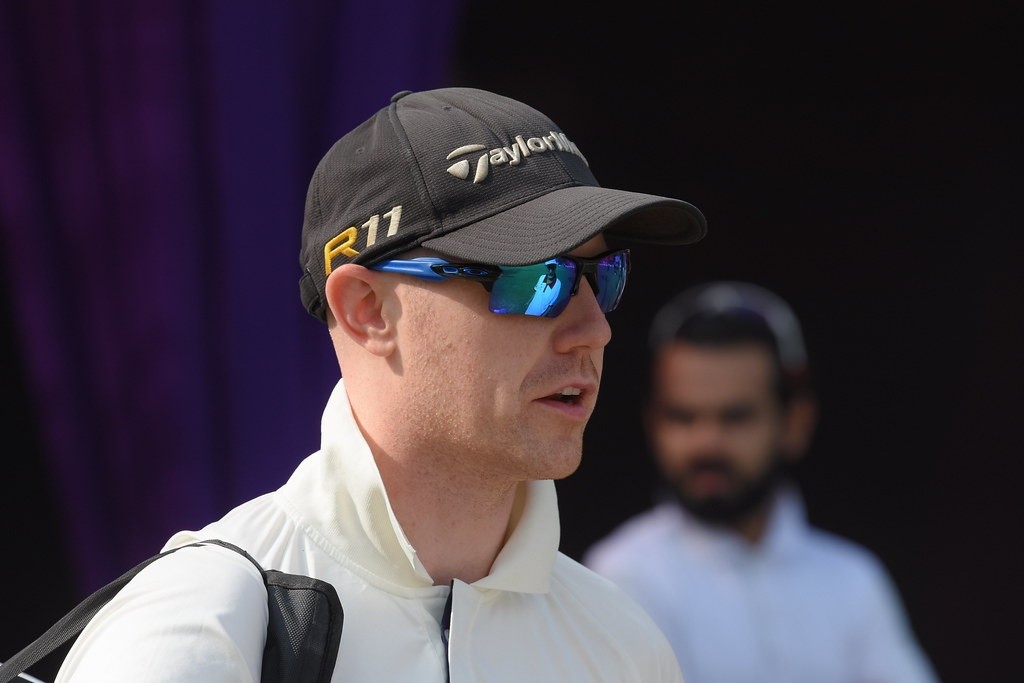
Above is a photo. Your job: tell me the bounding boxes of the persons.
[586,279,936,681]
[53,85,704,680]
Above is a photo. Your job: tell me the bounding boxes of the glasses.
[374,248,632,320]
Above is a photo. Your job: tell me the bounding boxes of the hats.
[298,87,709,325]
